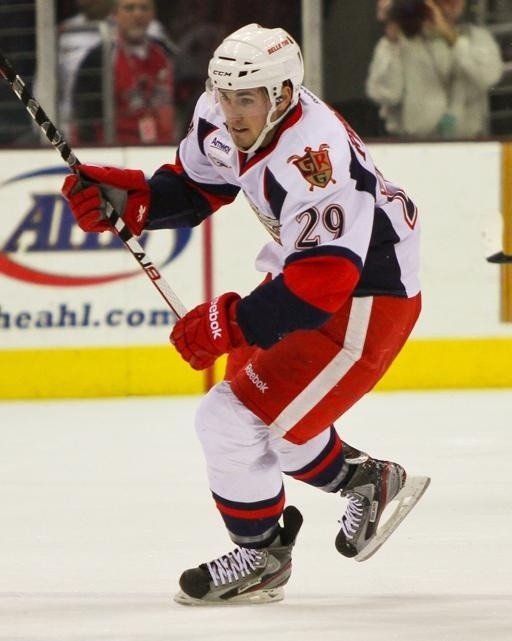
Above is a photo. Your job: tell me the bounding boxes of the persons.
[364,0,504,137]
[61,19,428,600]
[63,0,199,145]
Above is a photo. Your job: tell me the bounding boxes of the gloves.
[63,163,151,236]
[168,293,246,371]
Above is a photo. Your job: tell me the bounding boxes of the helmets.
[207,22,303,111]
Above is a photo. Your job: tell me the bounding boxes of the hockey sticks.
[482,210,511,262]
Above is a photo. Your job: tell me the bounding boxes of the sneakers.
[335,463,407,557]
[179,546,291,600]
